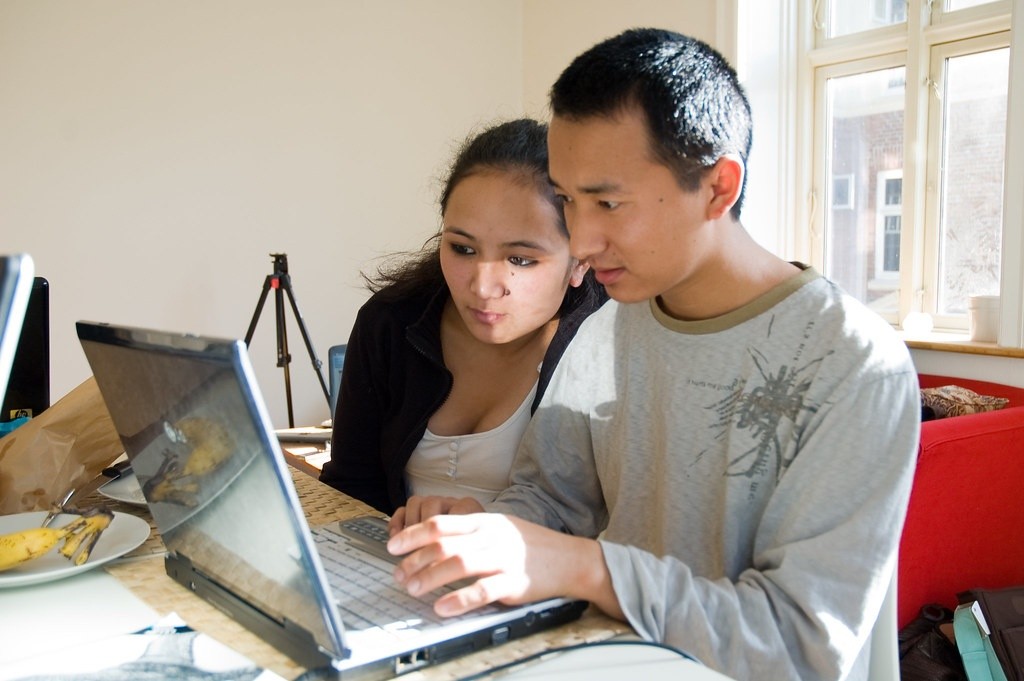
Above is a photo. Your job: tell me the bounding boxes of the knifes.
[64,460,135,510]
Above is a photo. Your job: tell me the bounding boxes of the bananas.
[141,418,233,502]
[0,504,115,572]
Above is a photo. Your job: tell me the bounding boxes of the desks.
[0,439,738,679]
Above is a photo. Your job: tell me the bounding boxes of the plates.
[96,451,152,507]
[0,510,149,589]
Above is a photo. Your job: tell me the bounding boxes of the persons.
[319,119,610,511]
[387,27,923,681]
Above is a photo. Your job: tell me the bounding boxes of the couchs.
[896,366,1024,636]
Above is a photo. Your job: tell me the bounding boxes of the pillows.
[915,383,1012,421]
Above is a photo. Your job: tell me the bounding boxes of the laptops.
[328,344,348,427]
[76,322,589,681]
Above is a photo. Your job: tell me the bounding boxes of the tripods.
[244,252,331,431]
